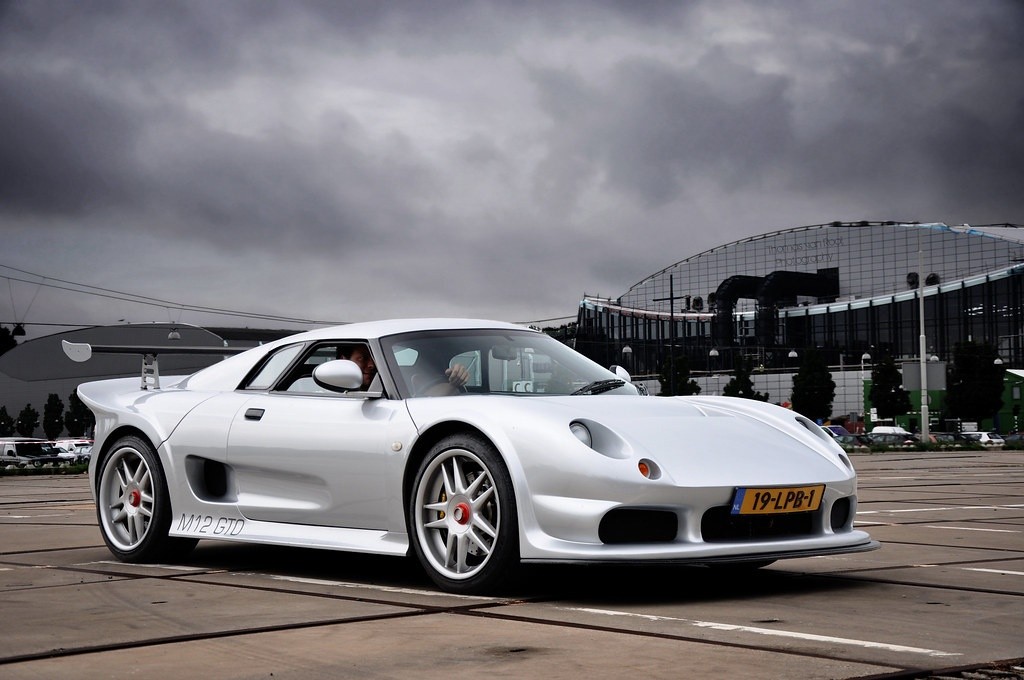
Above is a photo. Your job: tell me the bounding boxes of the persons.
[336,343,470,397]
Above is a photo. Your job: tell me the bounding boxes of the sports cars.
[62,318,882,598]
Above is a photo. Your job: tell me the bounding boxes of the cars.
[959,431,1005,448]
[50,440,92,464]
[905,432,982,448]
[820,425,869,449]
[866,426,913,443]
[0,437,75,468]
